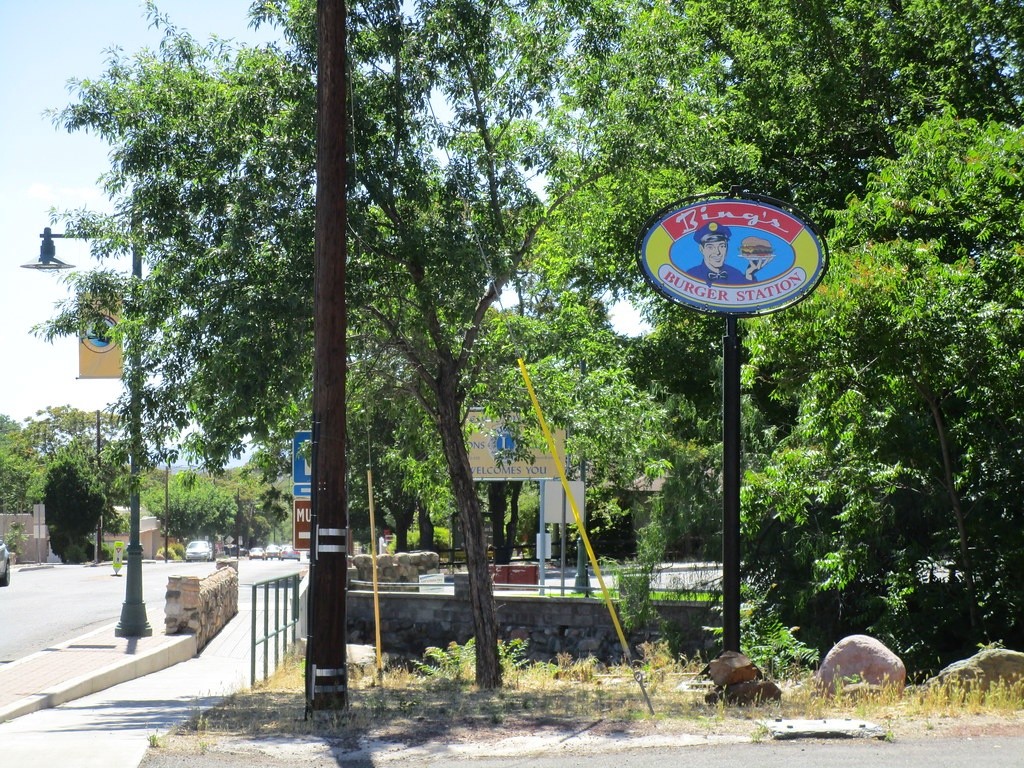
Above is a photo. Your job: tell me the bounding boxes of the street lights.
[18,209,153,638]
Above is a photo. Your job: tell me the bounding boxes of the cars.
[248,548,265,560]
[280,546,300,561]
[185,540,213,561]
[0,539,11,586]
[265,545,281,559]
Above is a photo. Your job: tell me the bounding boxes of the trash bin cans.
[491,564,540,591]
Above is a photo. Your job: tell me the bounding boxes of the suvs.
[225,543,247,557]
